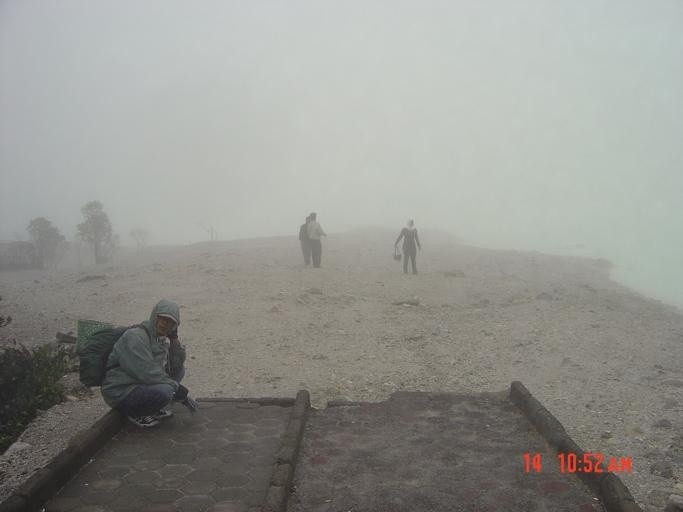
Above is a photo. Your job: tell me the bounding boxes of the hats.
[158,314,178,326]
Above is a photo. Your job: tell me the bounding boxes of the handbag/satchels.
[394,254,402,260]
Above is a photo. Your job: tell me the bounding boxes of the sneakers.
[128,410,172,427]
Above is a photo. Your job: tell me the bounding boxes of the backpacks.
[79,325,108,386]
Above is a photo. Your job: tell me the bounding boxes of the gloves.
[182,398,199,415]
[167,328,179,338]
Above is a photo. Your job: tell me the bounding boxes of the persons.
[394,219,422,274]
[101,299,198,427]
[299,212,327,268]
